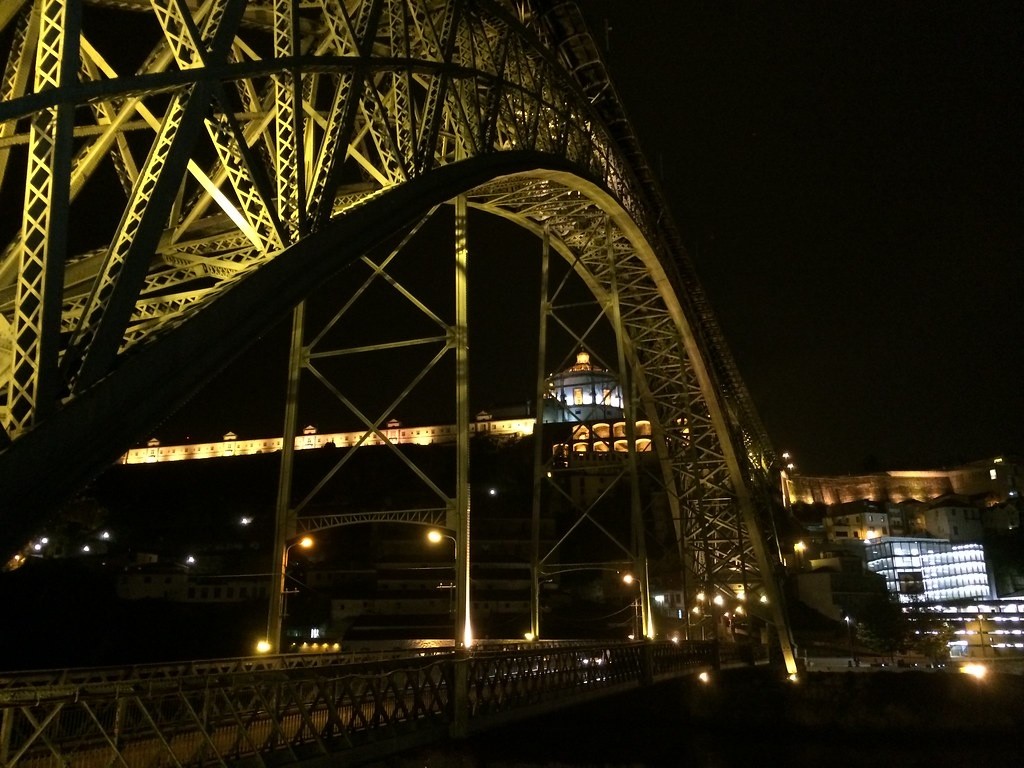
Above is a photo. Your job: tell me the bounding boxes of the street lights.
[844,616,856,666]
[977,615,987,657]
